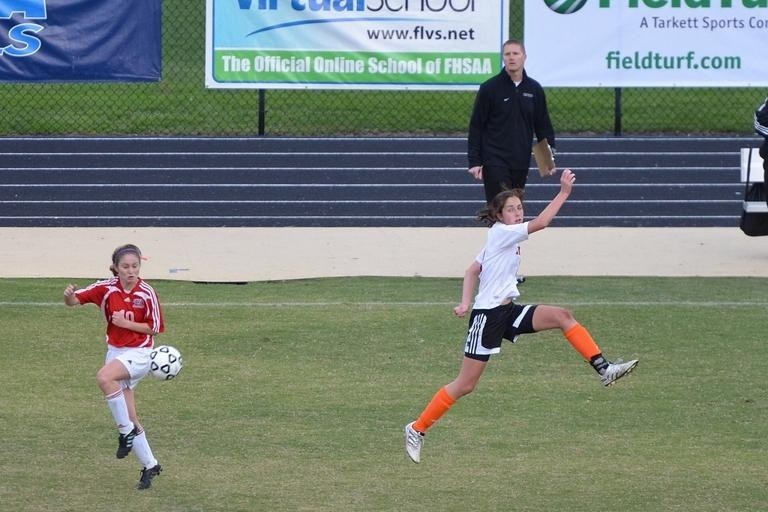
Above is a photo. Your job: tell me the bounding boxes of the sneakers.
[599,357,639,387]
[404,420,425,464]
[137,459,163,490]
[114,423,139,460]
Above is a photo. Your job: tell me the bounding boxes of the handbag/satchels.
[739,110,768,236]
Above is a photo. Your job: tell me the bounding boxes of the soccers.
[150,346,183,380]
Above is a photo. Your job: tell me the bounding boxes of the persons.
[402,170,640,463]
[466,37,556,285]
[62,243,165,490]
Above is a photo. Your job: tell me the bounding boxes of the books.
[532,138,555,178]
